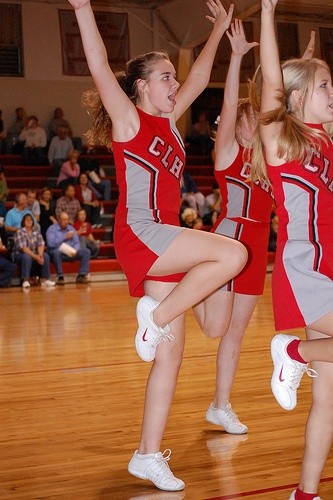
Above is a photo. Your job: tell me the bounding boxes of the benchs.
[0,145,217,272]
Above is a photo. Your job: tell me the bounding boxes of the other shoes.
[91,223,102,229]
[58,278,66,286]
[76,275,91,284]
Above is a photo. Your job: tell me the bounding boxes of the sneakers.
[270,334,319,410]
[288,489,322,500]
[134,295,176,362]
[127,449,185,491]
[41,279,56,286]
[204,401,249,435]
[22,281,30,288]
